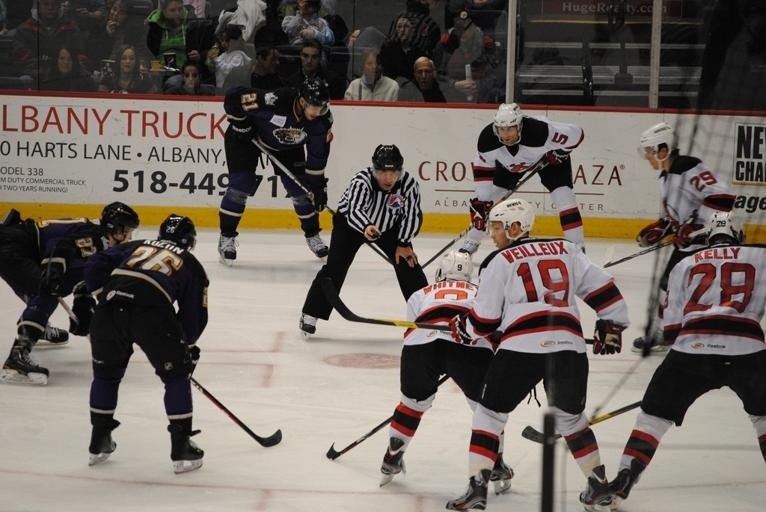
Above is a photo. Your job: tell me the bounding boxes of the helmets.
[100,202,140,241]
[491,102,523,146]
[705,209,744,246]
[297,79,329,119]
[639,122,679,162]
[159,213,198,251]
[371,145,404,182]
[487,198,536,242]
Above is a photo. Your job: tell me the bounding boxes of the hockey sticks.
[194,380,282,447]
[327,369,454,460]
[602,225,710,270]
[320,276,595,345]
[522,399,644,445]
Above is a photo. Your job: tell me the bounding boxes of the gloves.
[636,216,675,248]
[591,319,625,358]
[545,150,570,167]
[468,199,493,232]
[161,337,200,379]
[446,314,479,349]
[64,296,97,338]
[303,177,329,213]
[226,115,257,147]
[672,222,704,247]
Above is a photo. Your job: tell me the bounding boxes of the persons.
[344,46,400,102]
[96,44,159,95]
[400,56,448,102]
[249,46,288,90]
[284,37,334,101]
[630,120,744,353]
[588,4,642,65]
[661,0,708,68]
[0,201,141,386]
[388,12,429,80]
[323,13,360,100]
[13,0,96,81]
[606,209,766,512]
[298,141,430,340]
[88,0,142,59]
[145,0,221,88]
[214,23,264,88]
[378,41,425,102]
[438,8,507,102]
[458,101,586,259]
[163,60,216,97]
[68,1,109,44]
[217,75,334,268]
[281,0,335,74]
[33,45,99,91]
[379,251,515,497]
[445,198,615,512]
[83,213,210,475]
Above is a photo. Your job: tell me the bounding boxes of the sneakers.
[299,313,318,334]
[2,348,49,377]
[218,235,238,260]
[578,467,612,507]
[490,453,514,481]
[88,425,117,455]
[380,448,405,475]
[167,433,204,461]
[445,476,487,511]
[608,470,633,500]
[633,328,669,349]
[39,325,68,343]
[304,233,329,258]
